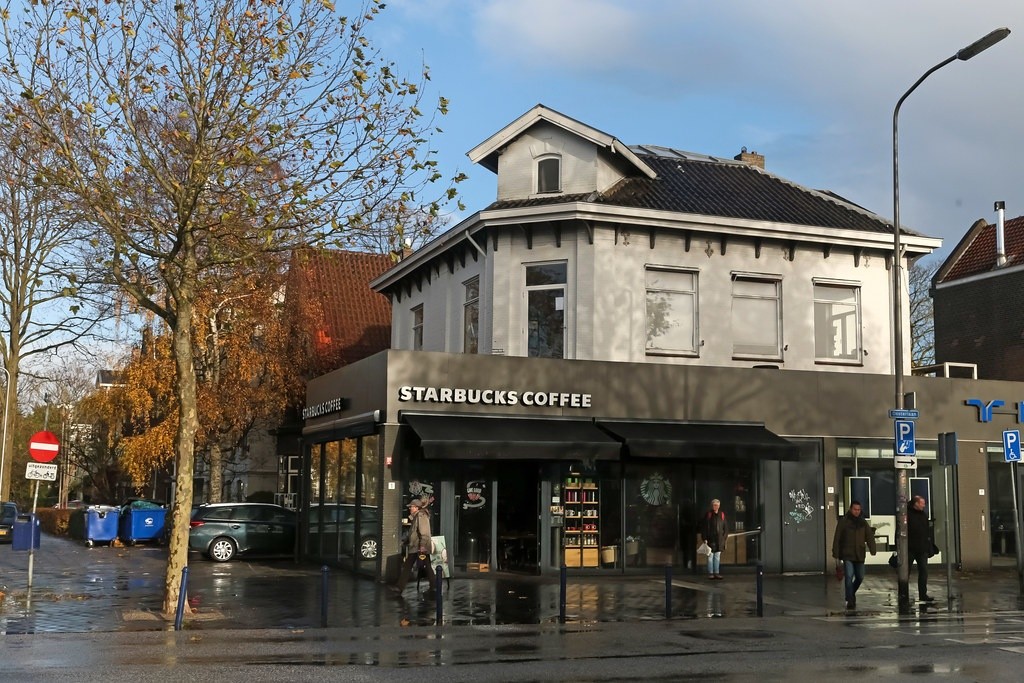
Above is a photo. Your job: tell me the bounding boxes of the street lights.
[890,27,1015,614]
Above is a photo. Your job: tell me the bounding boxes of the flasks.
[566,490,595,502]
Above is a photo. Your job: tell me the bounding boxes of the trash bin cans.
[121,509,168,546]
[82,510,122,547]
[10,513,40,550]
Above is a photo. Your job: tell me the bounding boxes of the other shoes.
[846,600,856,609]
[713,573,724,579]
[424,585,436,594]
[708,573,715,579]
[386,590,401,597]
[919,595,934,602]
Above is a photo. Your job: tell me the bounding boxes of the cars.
[307,501,378,561]
[51,501,88,510]
[188,502,302,562]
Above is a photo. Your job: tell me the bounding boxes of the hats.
[406,499,422,507]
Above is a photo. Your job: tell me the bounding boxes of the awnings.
[598,421,800,462]
[401,415,622,461]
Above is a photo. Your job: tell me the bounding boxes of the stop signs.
[28,431,60,463]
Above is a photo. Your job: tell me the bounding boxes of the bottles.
[578,534,597,544]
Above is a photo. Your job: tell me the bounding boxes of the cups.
[565,510,597,530]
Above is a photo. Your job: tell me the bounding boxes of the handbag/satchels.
[888,552,898,568]
[696,543,712,556]
[835,556,844,581]
[928,539,939,558]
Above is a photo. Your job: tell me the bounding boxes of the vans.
[0,501,18,545]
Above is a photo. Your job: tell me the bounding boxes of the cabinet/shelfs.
[563,472,602,569]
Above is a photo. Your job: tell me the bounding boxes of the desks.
[991,528,1014,556]
[486,534,537,569]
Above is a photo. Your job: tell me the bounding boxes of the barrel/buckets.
[601,546,618,563]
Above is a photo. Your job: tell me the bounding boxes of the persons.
[894,495,939,601]
[700,499,728,579]
[832,502,877,609]
[388,499,436,595]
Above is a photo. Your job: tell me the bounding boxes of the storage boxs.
[467,563,489,572]
